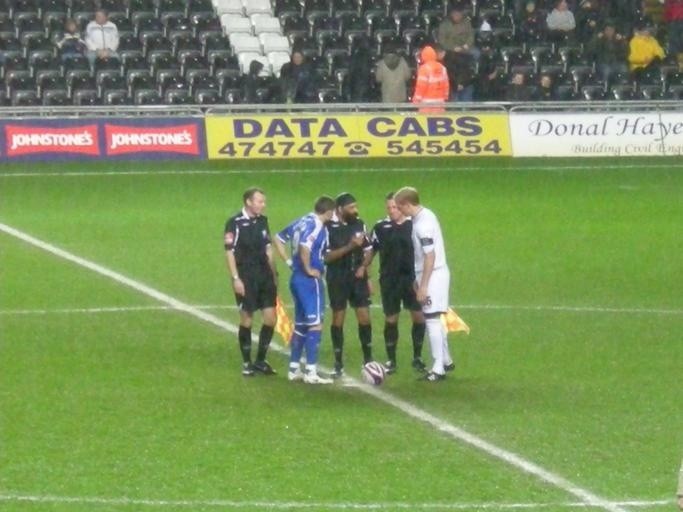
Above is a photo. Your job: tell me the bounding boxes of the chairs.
[0,0,683,113]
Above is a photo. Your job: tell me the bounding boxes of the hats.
[336,193,355,207]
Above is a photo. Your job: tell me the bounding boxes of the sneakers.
[242,360,254,376]
[416,370,446,383]
[361,360,367,370]
[303,368,334,384]
[328,366,346,380]
[444,363,455,372]
[288,367,306,382]
[251,359,280,376]
[383,359,400,373]
[411,357,430,373]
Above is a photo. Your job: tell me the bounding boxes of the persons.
[83,9,119,75]
[354,191,426,373]
[223,188,279,376]
[394,186,454,382]
[281,48,315,103]
[52,21,86,76]
[321,192,373,379]
[274,195,335,384]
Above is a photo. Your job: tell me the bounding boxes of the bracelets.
[347,242,354,250]
[231,275,239,280]
[361,263,368,268]
[286,259,292,266]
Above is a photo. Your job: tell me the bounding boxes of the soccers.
[360,361,385,386]
